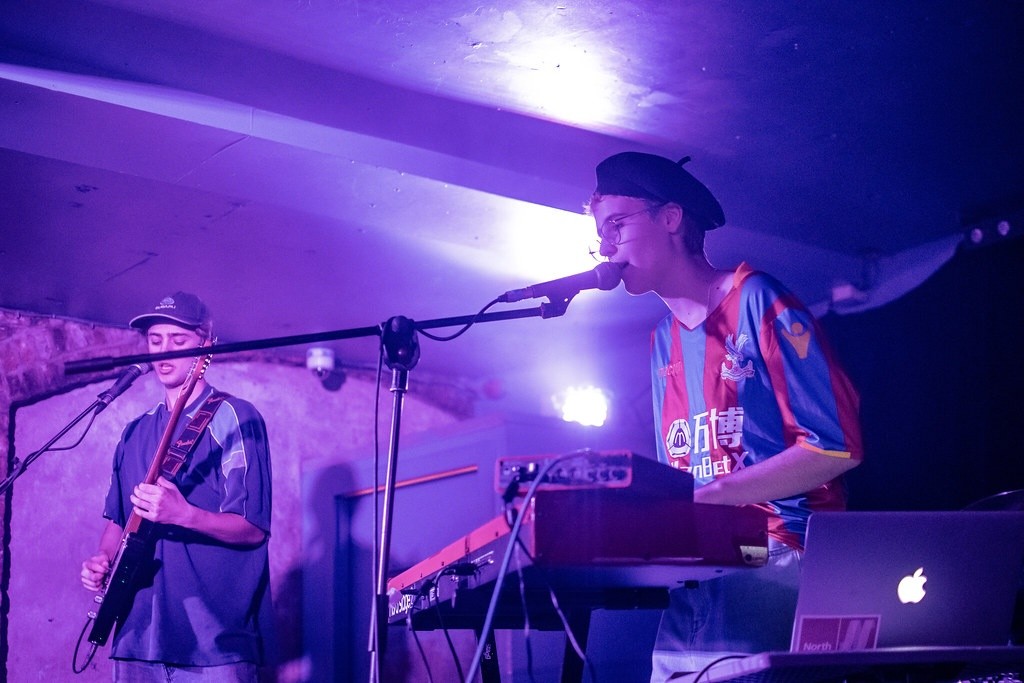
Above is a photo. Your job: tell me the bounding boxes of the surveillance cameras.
[307,349,335,380]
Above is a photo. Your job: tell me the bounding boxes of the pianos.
[387,490,770,626]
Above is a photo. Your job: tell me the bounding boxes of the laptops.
[789,510,1024,654]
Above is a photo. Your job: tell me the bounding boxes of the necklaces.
[706,268,717,319]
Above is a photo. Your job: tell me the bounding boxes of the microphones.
[94,362,149,416]
[498,262,623,302]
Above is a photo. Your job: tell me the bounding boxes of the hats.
[596,152,726,231]
[129,290,209,328]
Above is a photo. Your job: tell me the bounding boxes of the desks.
[672,643,1024,683]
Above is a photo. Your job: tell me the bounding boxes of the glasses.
[589,205,658,262]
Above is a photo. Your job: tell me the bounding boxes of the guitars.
[88,319,218,647]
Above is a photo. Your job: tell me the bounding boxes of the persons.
[588,152,864,683]
[80,291,273,683]
